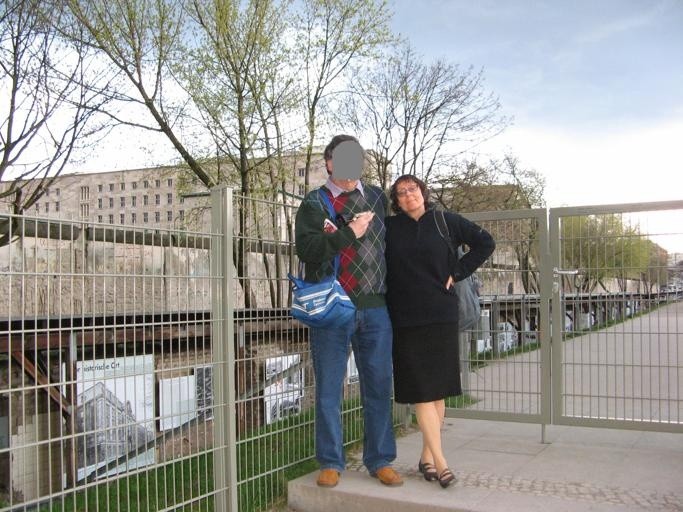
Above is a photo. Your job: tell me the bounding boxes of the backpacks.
[433,209,481,331]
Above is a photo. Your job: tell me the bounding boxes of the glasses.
[396,184,419,196]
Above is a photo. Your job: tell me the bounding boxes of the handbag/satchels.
[286,273,356,330]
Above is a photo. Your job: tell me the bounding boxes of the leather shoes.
[316,468,340,487]
[378,466,403,487]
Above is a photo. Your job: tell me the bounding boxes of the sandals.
[419,459,458,489]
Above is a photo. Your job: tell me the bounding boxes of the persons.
[384,173,496,490]
[294,136,404,489]
